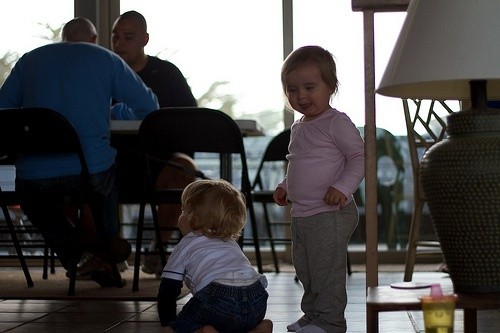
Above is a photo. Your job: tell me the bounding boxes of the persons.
[157,179,274,333]
[0,16,160,288]
[272,45,364,333]
[64,9,200,281]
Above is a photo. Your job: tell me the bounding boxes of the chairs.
[20,107,352,294]
[353,126,407,251]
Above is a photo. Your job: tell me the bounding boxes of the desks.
[109,119,267,138]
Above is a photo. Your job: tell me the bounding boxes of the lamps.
[376,0,500,308]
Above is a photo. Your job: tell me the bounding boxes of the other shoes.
[142,249,168,274]
[65,250,128,280]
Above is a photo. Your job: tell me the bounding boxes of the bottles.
[420,285,457,333]
